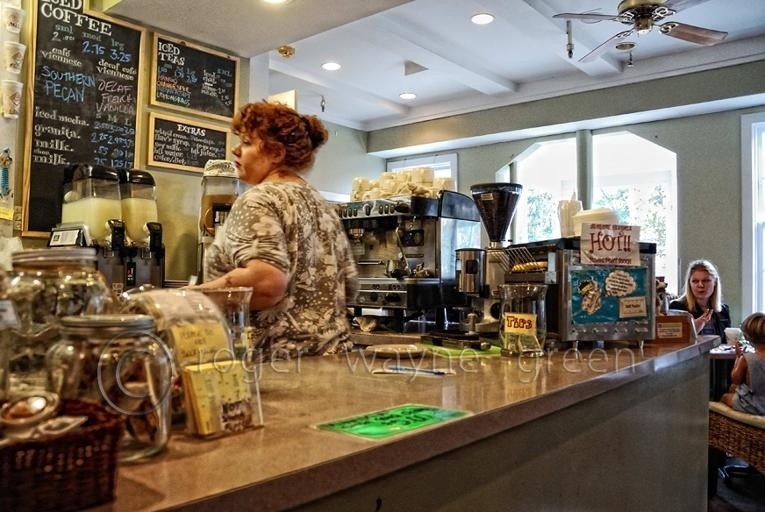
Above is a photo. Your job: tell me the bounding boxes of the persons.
[178,100,361,362]
[721,312,765,416]
[668,259,731,344]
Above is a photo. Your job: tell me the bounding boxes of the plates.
[364,342,425,360]
[571,207,620,238]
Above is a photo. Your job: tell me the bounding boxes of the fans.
[552,0,729,63]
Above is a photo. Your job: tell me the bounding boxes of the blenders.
[199,177,241,281]
[57,160,169,291]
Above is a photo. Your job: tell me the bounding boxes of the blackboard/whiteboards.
[150,31,241,123]
[147,112,232,174]
[20,0,146,239]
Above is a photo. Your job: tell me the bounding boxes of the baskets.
[0,396,126,512]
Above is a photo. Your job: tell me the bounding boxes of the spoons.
[386,365,454,377]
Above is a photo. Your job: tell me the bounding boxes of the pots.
[352,310,423,334]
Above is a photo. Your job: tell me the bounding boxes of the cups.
[3,40,26,74]
[351,166,456,202]
[1,79,25,120]
[4,3,25,34]
[496,283,551,358]
[724,327,743,352]
[179,286,254,364]
[557,198,583,238]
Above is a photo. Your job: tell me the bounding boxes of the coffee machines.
[326,190,489,309]
[442,183,563,342]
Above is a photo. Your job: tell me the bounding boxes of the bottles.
[0,391,61,434]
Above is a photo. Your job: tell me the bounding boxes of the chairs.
[707,401,765,501]
